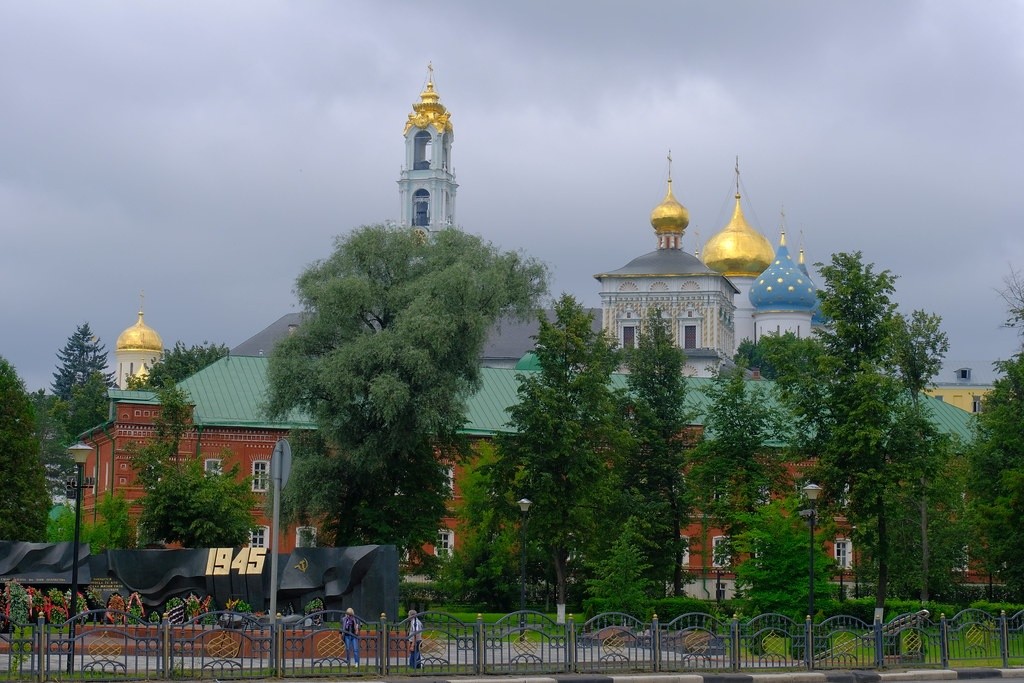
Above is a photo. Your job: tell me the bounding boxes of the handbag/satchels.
[354,619,359,634]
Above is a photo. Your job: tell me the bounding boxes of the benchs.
[886,655,919,666]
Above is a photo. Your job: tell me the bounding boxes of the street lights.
[803,483,821,670]
[516,497,532,643]
[66,439,94,677]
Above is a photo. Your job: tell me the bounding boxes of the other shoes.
[355,662,358,668]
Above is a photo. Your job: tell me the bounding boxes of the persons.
[408,609,424,668]
[339,607,366,668]
